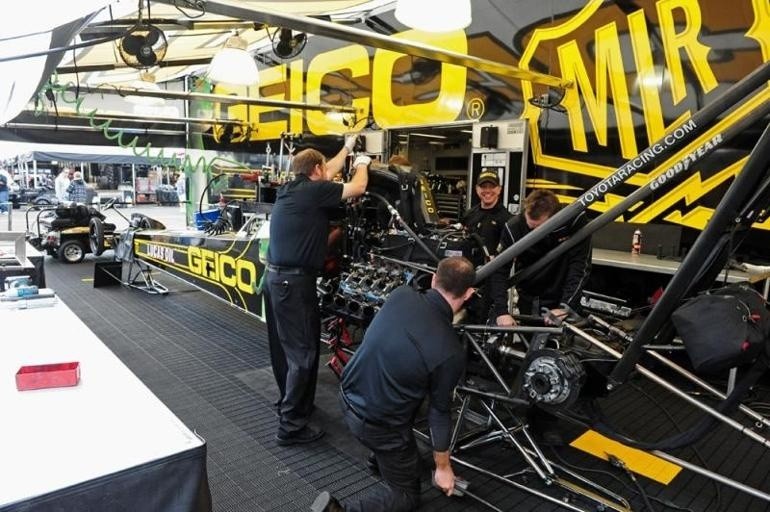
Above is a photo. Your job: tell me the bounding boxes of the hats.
[477,172,500,186]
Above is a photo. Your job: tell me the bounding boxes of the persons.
[261,136,369,446]
[492,191,590,327]
[54,167,73,202]
[306,257,475,511]
[461,172,508,264]
[66,172,93,204]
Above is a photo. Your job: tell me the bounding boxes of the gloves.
[353,157,373,167]
[346,133,361,151]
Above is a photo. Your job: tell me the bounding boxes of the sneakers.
[311,490,342,511]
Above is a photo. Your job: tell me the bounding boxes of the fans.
[119,23,168,71]
[273,29,308,58]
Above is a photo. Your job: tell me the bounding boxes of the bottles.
[632,228,642,254]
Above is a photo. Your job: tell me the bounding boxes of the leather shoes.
[274,421,327,447]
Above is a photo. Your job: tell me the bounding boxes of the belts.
[519,291,553,308]
[264,264,317,277]
[337,382,387,432]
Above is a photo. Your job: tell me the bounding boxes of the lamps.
[208,35,260,87]
[394,1,472,32]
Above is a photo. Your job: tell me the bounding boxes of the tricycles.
[42,198,168,264]
